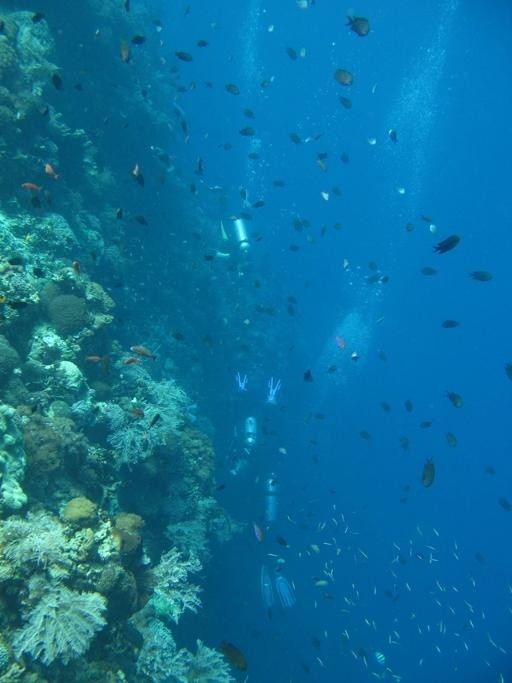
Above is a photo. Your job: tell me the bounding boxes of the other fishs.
[1,0,512,683]
